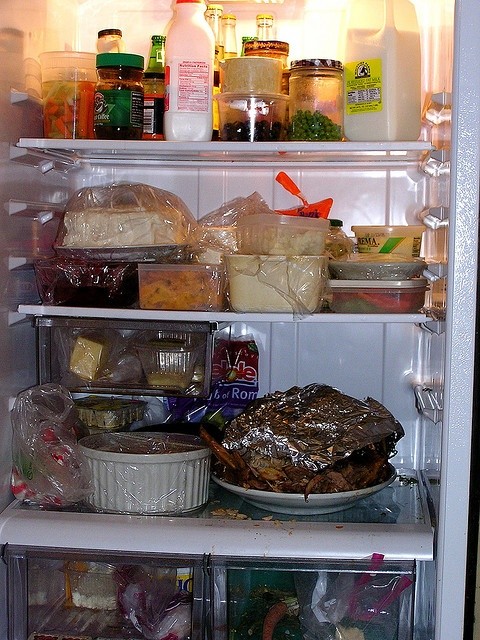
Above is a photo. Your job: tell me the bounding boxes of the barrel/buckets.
[344,0,422,142]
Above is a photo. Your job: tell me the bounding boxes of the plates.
[54,244,188,263]
[213,461,398,516]
[328,259,427,280]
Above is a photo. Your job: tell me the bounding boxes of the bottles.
[165,0,215,141]
[144,72,166,141]
[222,14,238,60]
[242,37,257,58]
[144,35,166,73]
[206,4,224,142]
[288,58,346,141]
[94,53,144,140]
[256,13,274,40]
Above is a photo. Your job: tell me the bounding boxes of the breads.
[62,204,184,247]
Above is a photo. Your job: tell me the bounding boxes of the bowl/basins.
[79,428,211,513]
